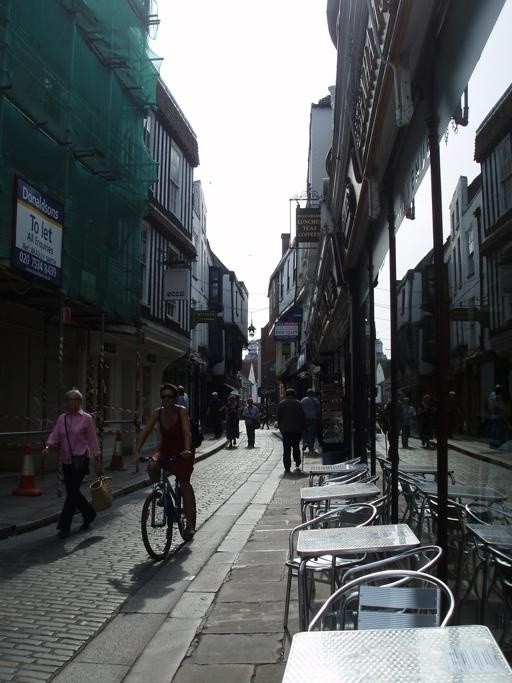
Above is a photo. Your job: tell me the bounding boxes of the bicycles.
[130,446,197,561]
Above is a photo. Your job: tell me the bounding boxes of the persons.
[174,384,191,407]
[128,382,198,536]
[485,384,508,449]
[276,387,306,471]
[217,394,241,448]
[300,387,321,455]
[41,388,105,538]
[205,391,225,439]
[399,397,417,449]
[415,394,436,449]
[242,397,261,448]
[259,412,270,429]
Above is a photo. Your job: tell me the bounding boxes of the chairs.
[283,456,455,632]
[379,457,512,653]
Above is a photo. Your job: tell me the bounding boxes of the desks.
[281,623,512,682]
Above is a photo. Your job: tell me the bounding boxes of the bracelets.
[46,445,49,448]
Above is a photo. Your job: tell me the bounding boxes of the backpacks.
[157,405,204,449]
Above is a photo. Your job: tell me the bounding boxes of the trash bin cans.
[323,437,345,465]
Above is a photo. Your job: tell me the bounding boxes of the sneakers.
[302,444,316,454]
[80,514,96,530]
[184,521,197,536]
[284,467,301,474]
[228,441,255,448]
[57,527,70,537]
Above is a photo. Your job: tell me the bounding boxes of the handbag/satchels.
[88,476,113,512]
[70,454,91,478]
